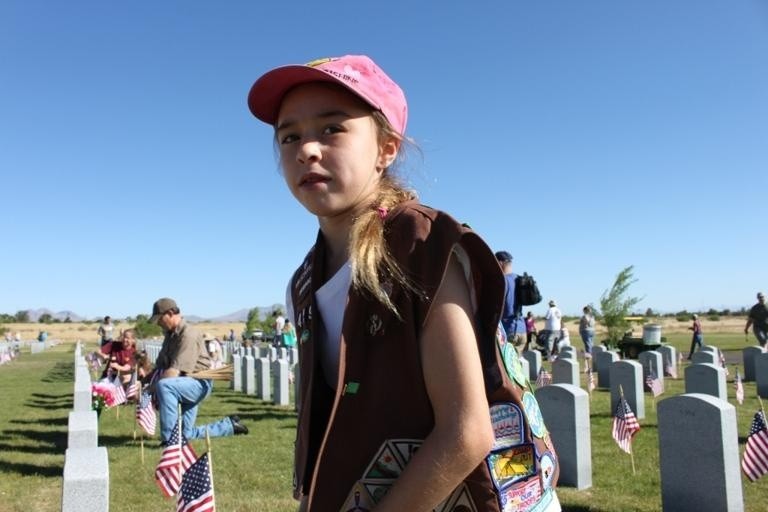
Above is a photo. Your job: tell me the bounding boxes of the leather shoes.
[227,415,248,435]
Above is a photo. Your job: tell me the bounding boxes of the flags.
[534,348,744,405]
[611,397,640,456]
[175,451,215,512]
[741,408,768,483]
[154,416,199,497]
[85,351,156,436]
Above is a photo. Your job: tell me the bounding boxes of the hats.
[496,252,513,263]
[148,298,178,322]
[246,53,409,140]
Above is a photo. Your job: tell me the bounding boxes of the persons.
[95,329,138,384]
[141,298,248,447]
[3,327,12,342]
[36,328,48,342]
[203,311,298,369]
[247,54,563,512]
[97,316,116,347]
[687,313,703,360]
[744,292,768,353]
[14,332,20,352]
[494,252,594,361]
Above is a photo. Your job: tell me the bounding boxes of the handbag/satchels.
[515,271,541,304]
